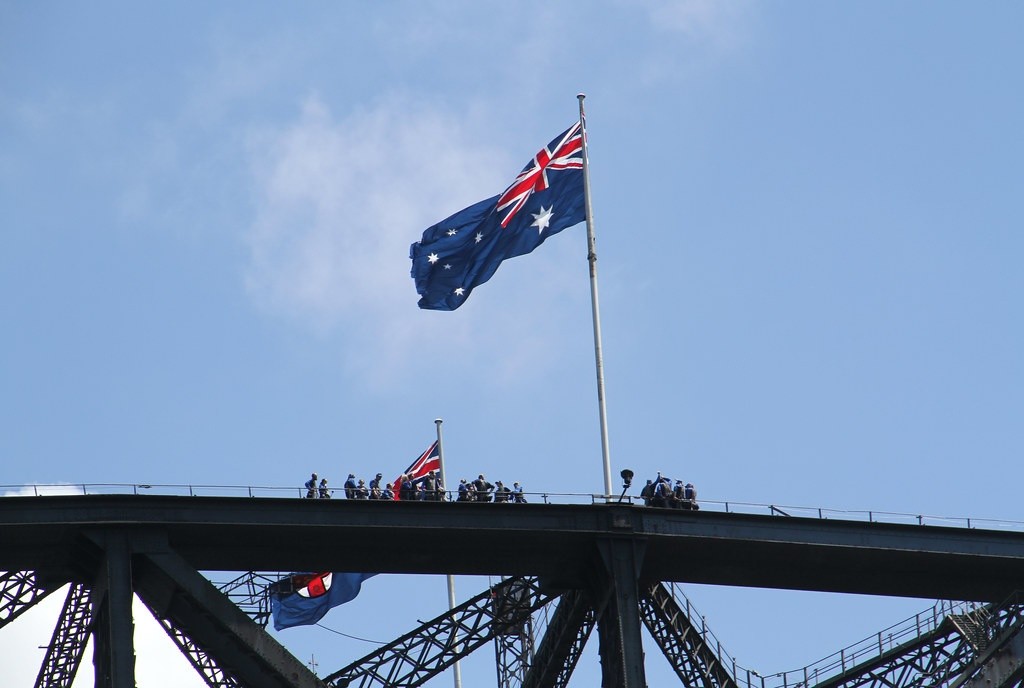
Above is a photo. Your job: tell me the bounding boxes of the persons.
[458,474,527,503]
[319,479,331,498]
[641,471,699,510]
[345,471,443,500]
[305,472,317,498]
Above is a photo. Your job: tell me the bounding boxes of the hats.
[495,481,503,485]
[376,473,382,477]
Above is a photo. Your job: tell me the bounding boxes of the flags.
[410,116,587,310]
[269,572,375,631]
[392,440,442,500]
